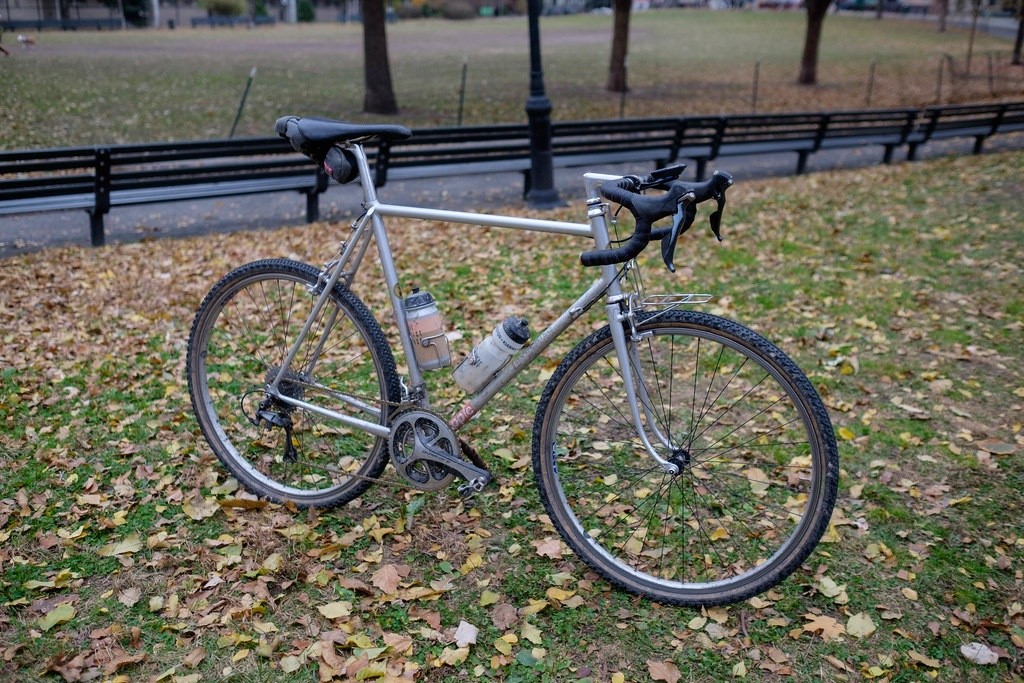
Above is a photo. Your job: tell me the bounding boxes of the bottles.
[451,316,531,395]
[404,286,451,373]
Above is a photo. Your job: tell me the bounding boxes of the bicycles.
[187,116,841,608]
